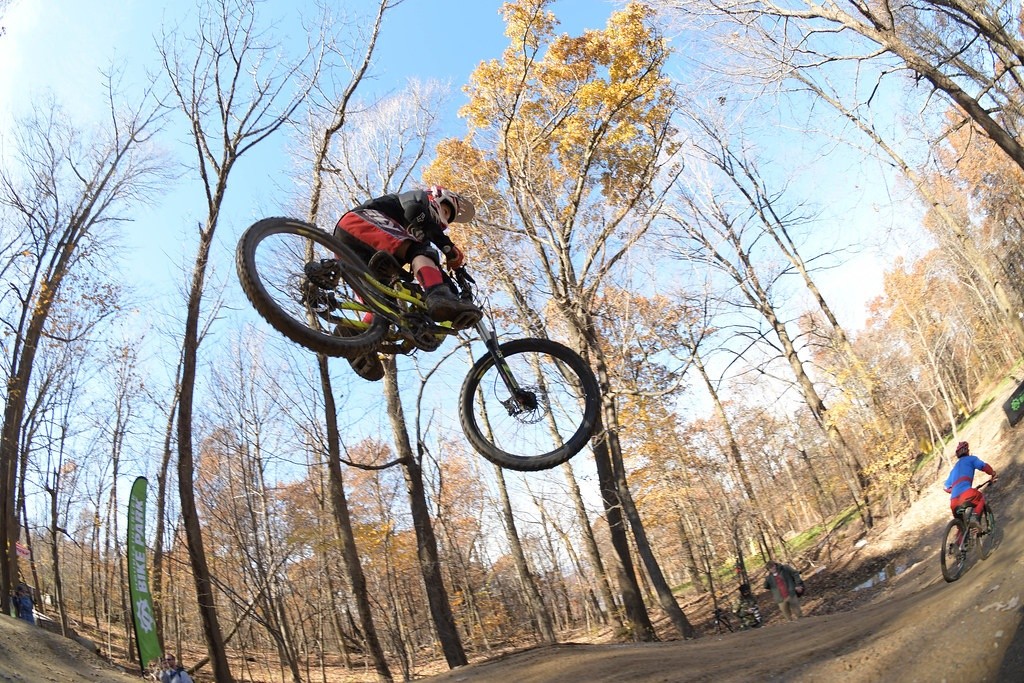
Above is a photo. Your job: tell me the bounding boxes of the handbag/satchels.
[792,579,805,597]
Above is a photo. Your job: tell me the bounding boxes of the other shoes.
[969,523,983,531]
[334,325,385,381]
[423,283,484,322]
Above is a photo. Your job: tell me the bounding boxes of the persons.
[733,585,761,631]
[332,185,483,382]
[145,653,194,683]
[762,560,804,621]
[942,441,999,561]
[13,587,35,625]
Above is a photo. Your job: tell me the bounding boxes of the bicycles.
[939,476,1000,584]
[233,215,604,471]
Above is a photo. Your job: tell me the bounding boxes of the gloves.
[446,246,463,272]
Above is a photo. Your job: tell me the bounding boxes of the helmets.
[427,184,475,230]
[956,441,969,458]
[16,584,26,592]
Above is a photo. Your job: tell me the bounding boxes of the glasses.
[166,658,174,661]
